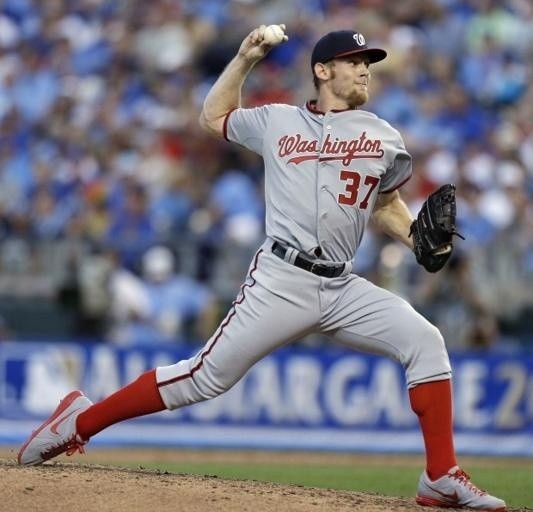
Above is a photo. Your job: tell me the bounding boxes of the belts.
[270,242,345,280]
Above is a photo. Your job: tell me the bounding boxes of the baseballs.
[263,24,284,46]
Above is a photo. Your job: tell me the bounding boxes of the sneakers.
[15,389,99,468]
[414,464,507,512]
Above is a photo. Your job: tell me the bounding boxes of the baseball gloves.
[408,184,464,273]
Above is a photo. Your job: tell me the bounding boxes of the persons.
[0,0,532,349]
[16,21,507,510]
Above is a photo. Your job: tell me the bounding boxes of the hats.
[309,27,387,68]
